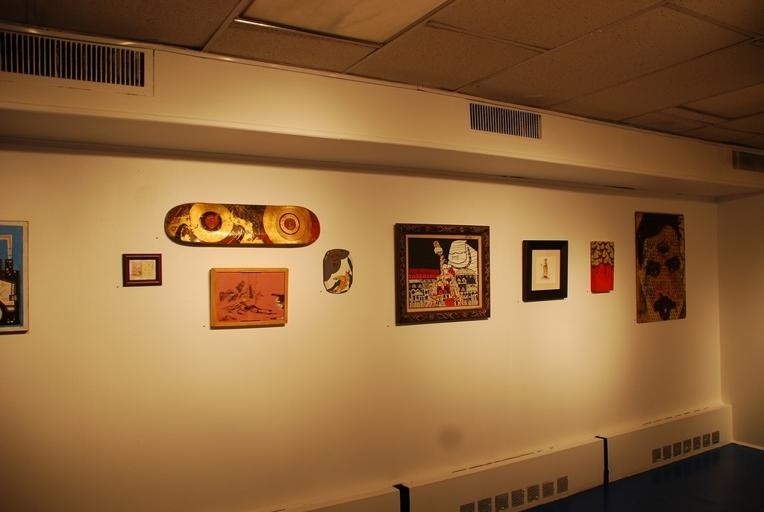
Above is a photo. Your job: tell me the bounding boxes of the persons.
[637,213,686,323]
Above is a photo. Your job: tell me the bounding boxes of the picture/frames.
[211,268,288,328]
[521,239,568,302]
[393,223,493,323]
[123,254,162,286]
[0,220,30,334]
